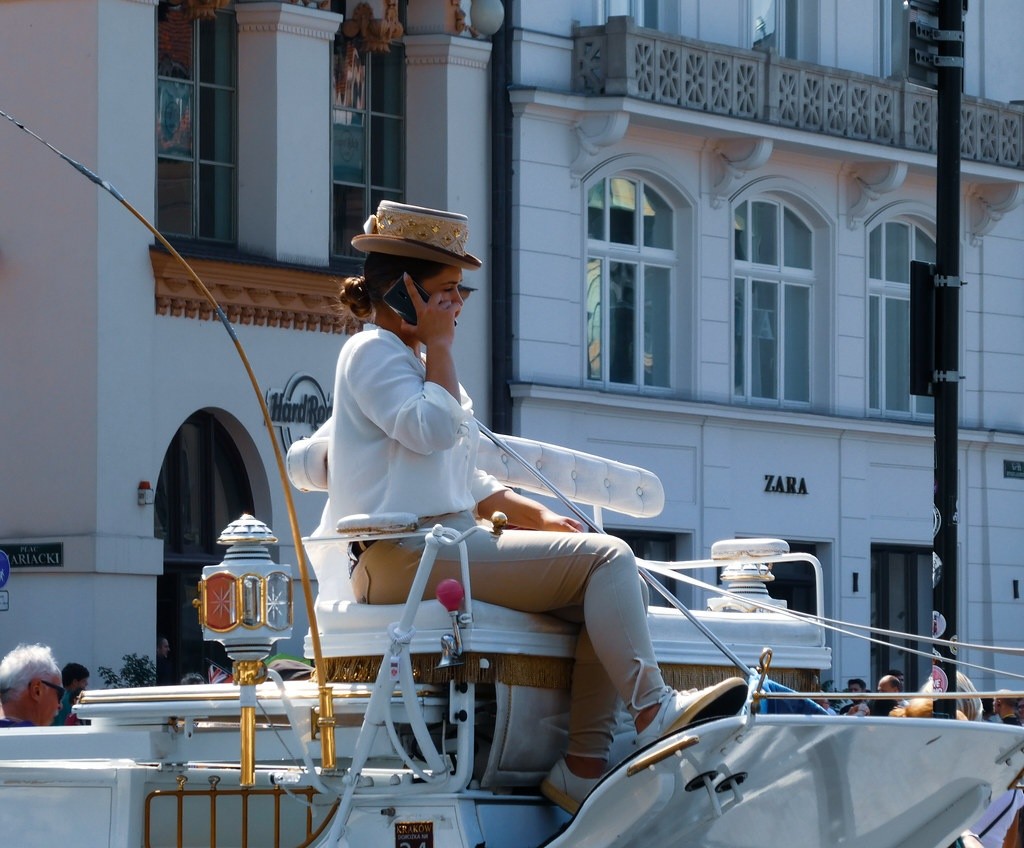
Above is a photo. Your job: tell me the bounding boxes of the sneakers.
[542,757,599,817]
[630,657,748,748]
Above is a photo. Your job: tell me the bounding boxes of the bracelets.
[959,833,981,843]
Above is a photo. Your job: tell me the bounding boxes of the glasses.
[1017,704,1024,710]
[28,679,65,704]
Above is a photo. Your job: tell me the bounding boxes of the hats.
[263,658,316,681]
[351,198,483,271]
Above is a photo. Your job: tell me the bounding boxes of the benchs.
[284,415,833,794]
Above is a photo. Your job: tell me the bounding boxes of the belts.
[347,539,378,561]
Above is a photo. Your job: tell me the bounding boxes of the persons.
[328,201,748,816]
[0,637,208,728]
[821,671,1024,848]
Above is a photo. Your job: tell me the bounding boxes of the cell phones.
[384,276,458,327]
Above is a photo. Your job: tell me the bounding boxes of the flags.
[206,663,233,683]
